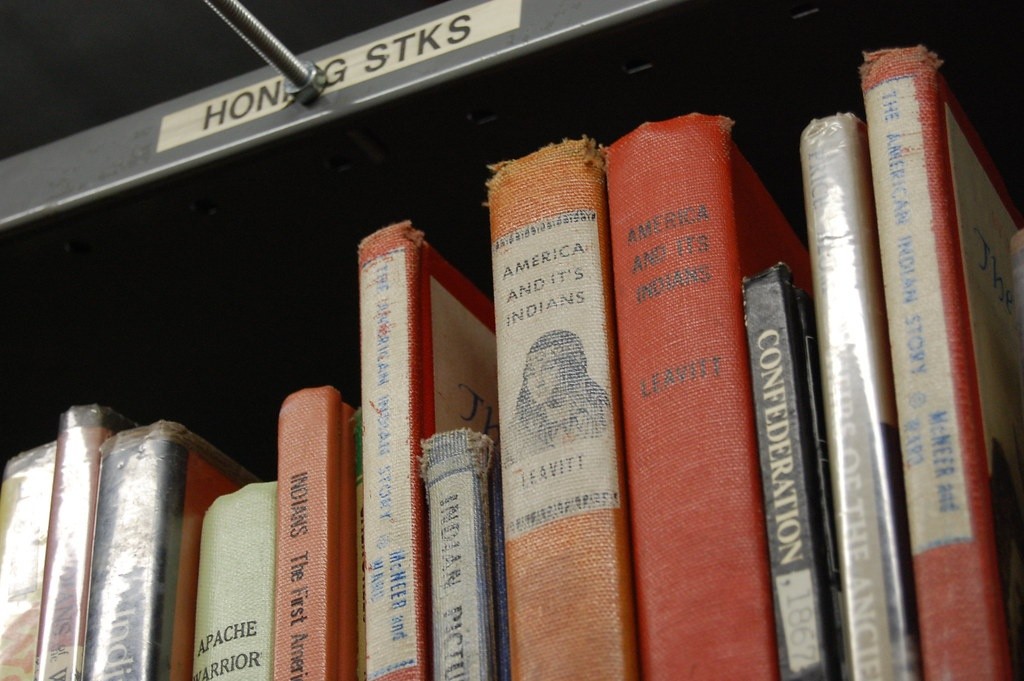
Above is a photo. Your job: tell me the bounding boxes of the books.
[0,405,262,681]
[604,112,816,680]
[801,112,922,681]
[276,386,358,680]
[358,220,499,680]
[862,45,1024,680]
[420,428,509,681]
[192,482,277,681]
[486,137,642,681]
[745,263,847,680]
[352,409,367,681]
